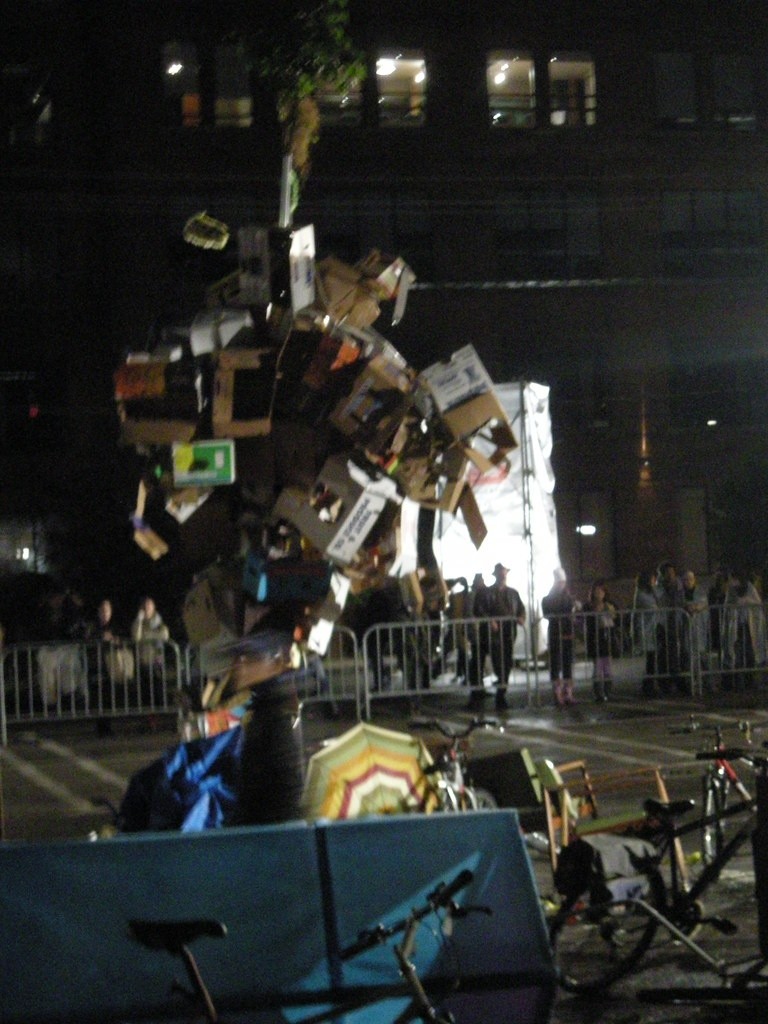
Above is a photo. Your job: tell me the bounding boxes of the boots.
[553,685,567,706]
[564,683,584,705]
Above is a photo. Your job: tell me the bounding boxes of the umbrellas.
[299,721,447,822]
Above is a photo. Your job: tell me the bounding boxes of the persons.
[34,591,170,734]
[115,670,299,837]
[544,561,768,707]
[346,563,526,713]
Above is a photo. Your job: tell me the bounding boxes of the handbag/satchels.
[105,639,135,683]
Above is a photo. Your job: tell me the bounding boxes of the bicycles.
[405,716,505,813]
[549,743,767,996]
[668,714,768,877]
[126,868,495,1024]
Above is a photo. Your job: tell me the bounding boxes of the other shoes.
[605,689,619,700]
[494,694,509,710]
[593,691,609,702]
[467,690,485,710]
